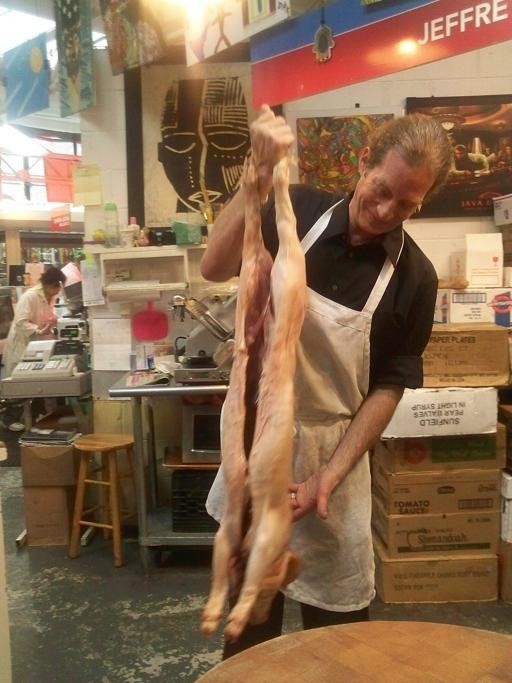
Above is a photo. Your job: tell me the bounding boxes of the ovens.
[180,403,225,465]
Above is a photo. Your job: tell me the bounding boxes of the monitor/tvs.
[9,264,25,286]
[0,293,15,338]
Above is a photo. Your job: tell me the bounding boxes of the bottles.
[128,217,140,237]
[103,202,121,249]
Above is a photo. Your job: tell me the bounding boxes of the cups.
[119,228,137,248]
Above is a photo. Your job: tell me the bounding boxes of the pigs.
[200,154,303,642]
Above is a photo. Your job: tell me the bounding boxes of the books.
[127,371,171,389]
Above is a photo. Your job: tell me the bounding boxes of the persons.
[445,127,512,177]
[198,103,458,659]
[0,267,68,433]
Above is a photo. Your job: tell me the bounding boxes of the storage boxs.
[371,422,506,474]
[370,493,500,560]
[371,529,498,605]
[434,288,496,324]
[422,322,512,387]
[371,455,501,516]
[499,472,511,544]
[19,432,81,547]
[499,539,511,606]
[380,388,498,440]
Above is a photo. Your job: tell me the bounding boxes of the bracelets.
[240,183,268,204]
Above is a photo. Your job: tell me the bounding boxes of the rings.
[291,492,296,499]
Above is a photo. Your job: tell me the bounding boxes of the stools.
[66,431,142,568]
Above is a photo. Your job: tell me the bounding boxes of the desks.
[107,369,230,574]
[191,619,511,683]
